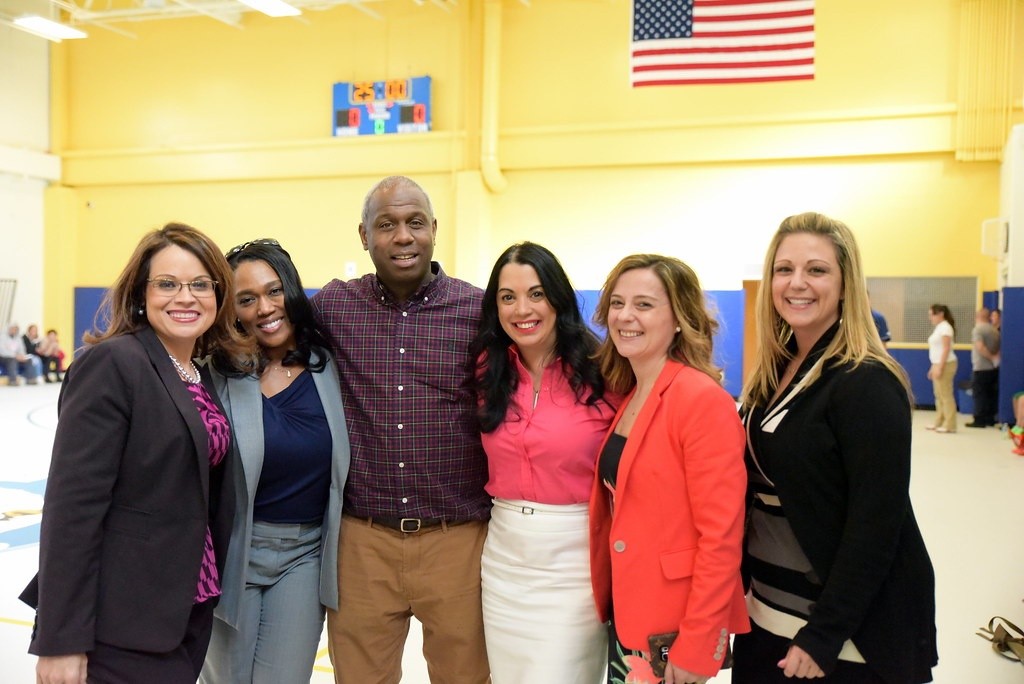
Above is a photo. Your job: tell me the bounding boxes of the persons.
[586,254,751,684]
[0,324,64,386]
[965,307,1000,428]
[730,213,938,684]
[870,310,891,350]
[309,176,492,684]
[457,241,636,684]
[17,221,256,684]
[197,239,350,684]
[926,304,959,434]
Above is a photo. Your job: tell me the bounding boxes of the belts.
[491,498,589,516]
[352,516,455,533]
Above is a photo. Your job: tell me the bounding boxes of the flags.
[630,0,815,89]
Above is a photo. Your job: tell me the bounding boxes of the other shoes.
[926,424,938,430]
[966,422,985,428]
[935,427,957,433]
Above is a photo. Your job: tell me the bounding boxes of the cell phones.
[648,631,734,677]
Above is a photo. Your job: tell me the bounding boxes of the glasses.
[146,278,218,297]
[224,238,284,260]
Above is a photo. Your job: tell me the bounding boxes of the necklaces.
[269,366,293,377]
[533,385,540,409]
[169,356,201,384]
